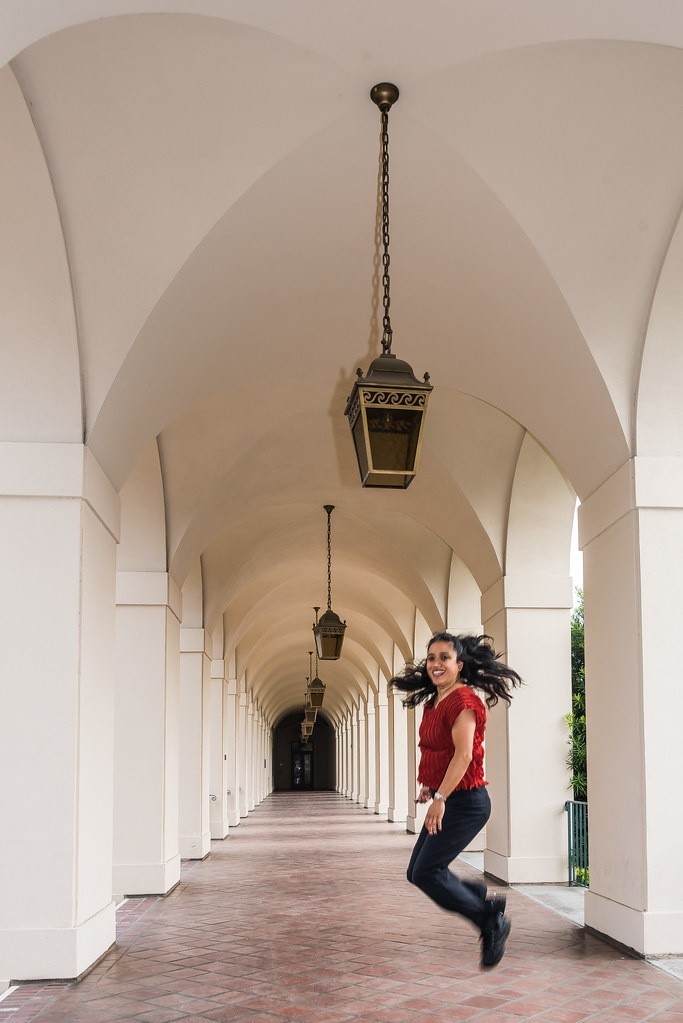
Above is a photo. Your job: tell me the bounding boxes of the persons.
[387,632,525,972]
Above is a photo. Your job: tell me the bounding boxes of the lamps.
[300,652,319,739]
[307,607,326,709]
[312,505,347,662]
[344,82,433,491]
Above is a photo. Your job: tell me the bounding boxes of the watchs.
[434,791,447,802]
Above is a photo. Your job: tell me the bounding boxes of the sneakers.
[480,913,511,967]
[484,899,506,914]
